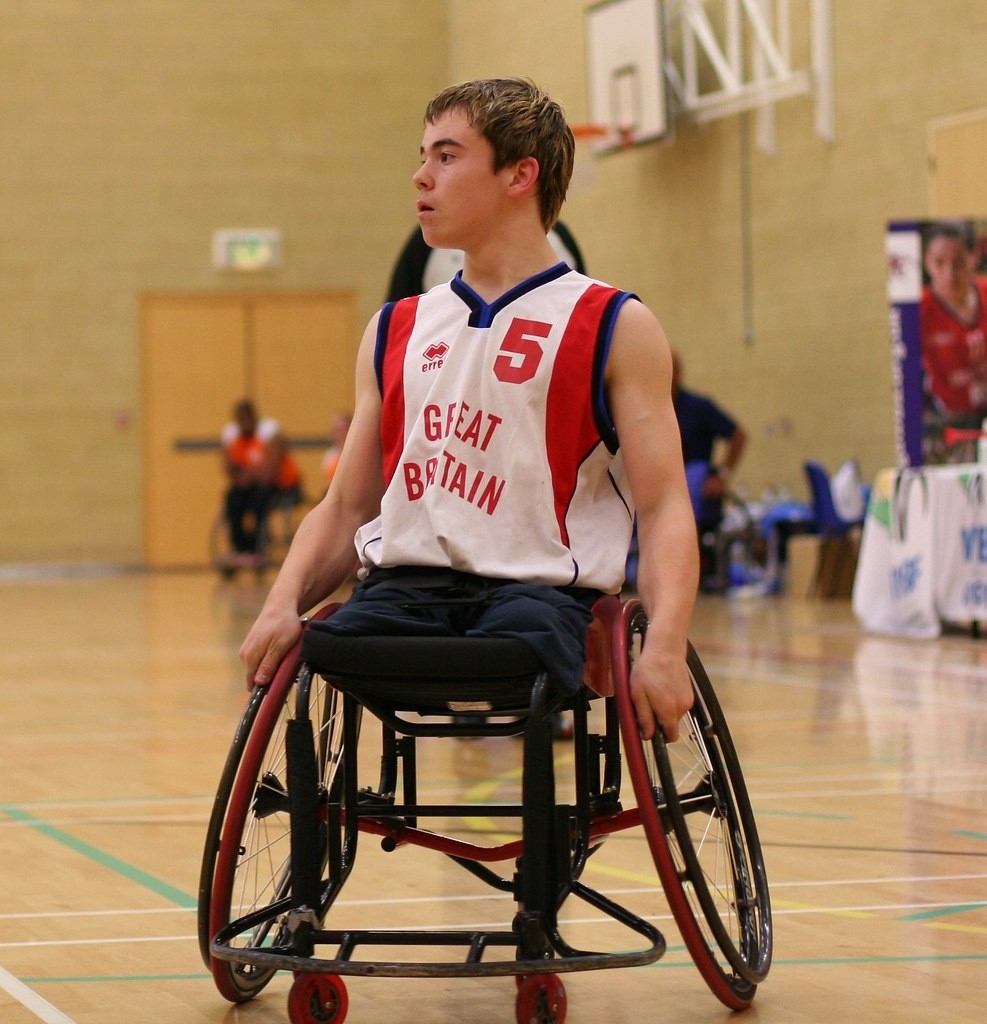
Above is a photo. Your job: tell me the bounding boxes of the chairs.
[800,459,872,601]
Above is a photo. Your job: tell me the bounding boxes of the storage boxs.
[788,530,859,598]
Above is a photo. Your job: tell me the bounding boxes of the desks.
[849,462,987,642]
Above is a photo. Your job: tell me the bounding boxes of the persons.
[220,401,305,582]
[920,219,987,447]
[624,351,746,586]
[238,79,699,743]
